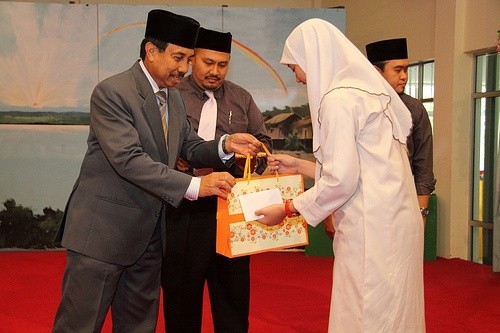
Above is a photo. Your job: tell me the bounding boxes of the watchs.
[285,199,301,218]
[420,207,429,217]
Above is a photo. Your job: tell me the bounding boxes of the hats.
[196,26,232,54]
[145,9,200,50]
[365,37,408,62]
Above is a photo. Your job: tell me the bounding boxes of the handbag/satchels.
[214,142,310,259]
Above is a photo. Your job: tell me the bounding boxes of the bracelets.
[222,136,233,155]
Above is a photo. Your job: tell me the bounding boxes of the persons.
[365,38,435,225]
[51,9,261,333]
[254,17,425,333]
[162,26,272,333]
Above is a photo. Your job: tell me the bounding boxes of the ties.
[155,91,170,152]
